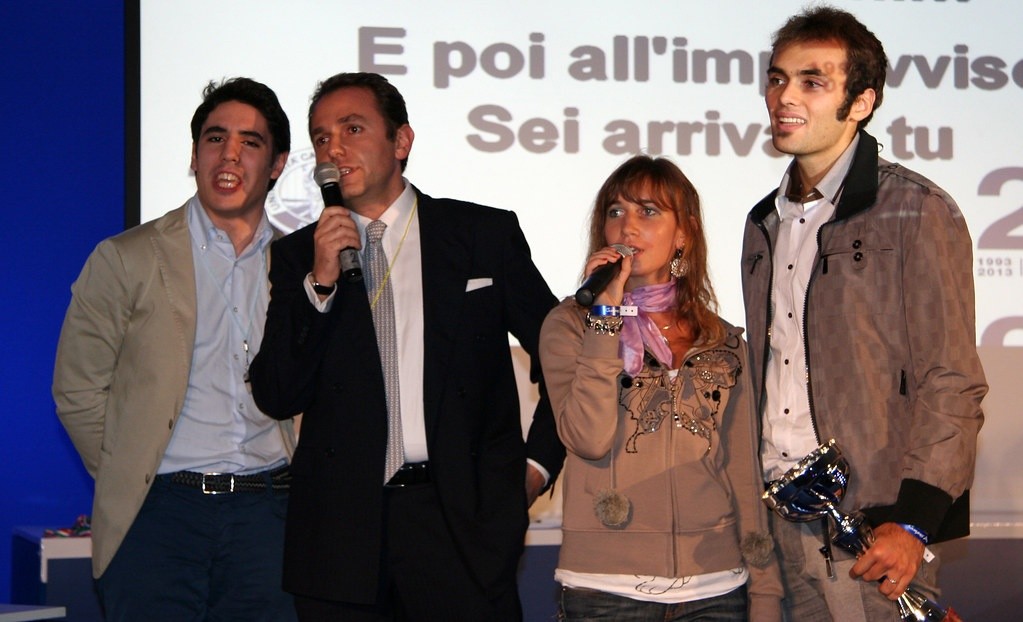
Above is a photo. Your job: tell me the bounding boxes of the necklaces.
[794,191,816,201]
[200,253,266,380]
[659,318,676,332]
[370,195,416,310]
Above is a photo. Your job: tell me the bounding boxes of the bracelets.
[589,304,620,317]
[903,523,930,546]
[584,312,623,336]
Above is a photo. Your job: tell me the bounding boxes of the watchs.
[308,273,335,295]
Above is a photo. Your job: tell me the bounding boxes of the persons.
[50,77,301,622]
[538,152,786,622]
[740,2,989,622]
[251,74,569,622]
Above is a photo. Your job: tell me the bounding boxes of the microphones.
[574,243,634,307]
[313,162,363,281]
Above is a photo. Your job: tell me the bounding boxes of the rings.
[888,578,896,584]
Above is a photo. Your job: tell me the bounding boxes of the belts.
[154,466,294,496]
[386,461,434,487]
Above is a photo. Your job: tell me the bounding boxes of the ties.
[360,219,407,487]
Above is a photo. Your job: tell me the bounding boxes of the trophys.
[760,438,947,622]
